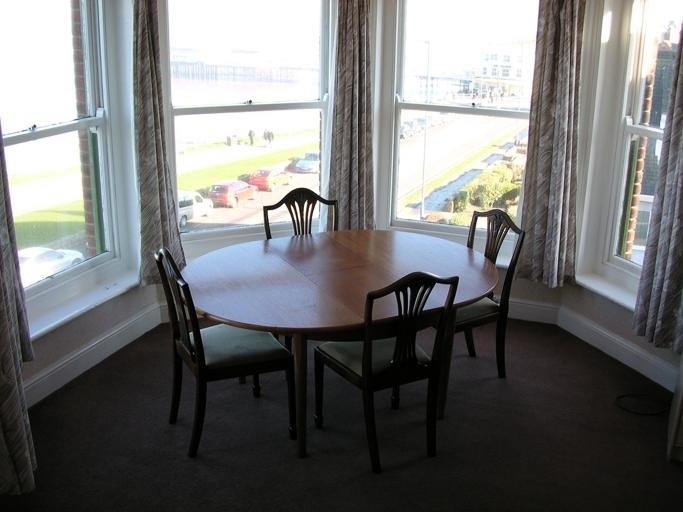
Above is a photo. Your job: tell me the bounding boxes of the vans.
[175,191,214,229]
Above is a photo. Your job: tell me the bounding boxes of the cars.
[250,166,291,191]
[296,152,320,174]
[208,181,257,207]
[14,245,84,292]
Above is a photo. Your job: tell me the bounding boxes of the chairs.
[432,210,528,380]
[313,271,459,474]
[152,247,302,458]
[263,187,339,374]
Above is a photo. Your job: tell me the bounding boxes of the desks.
[178,230,499,459]
